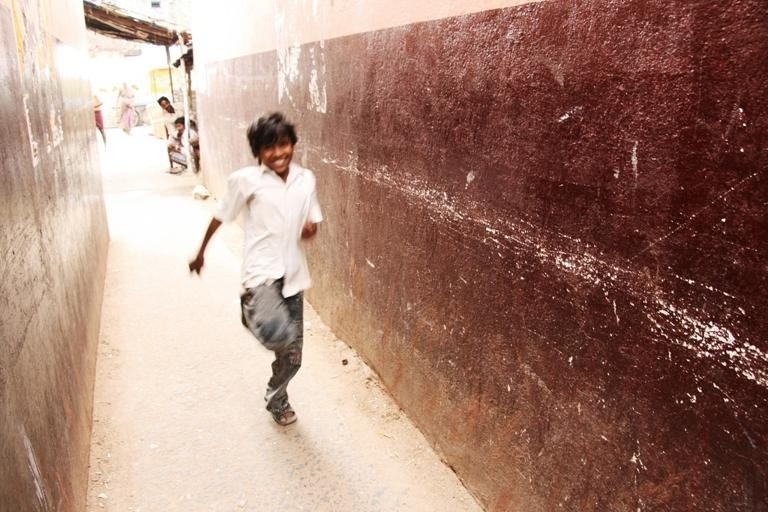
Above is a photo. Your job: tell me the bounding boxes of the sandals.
[272,405,297,426]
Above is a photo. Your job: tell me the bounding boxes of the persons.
[93,95,107,154]
[114,80,136,135]
[156,96,201,174]
[188,111,324,427]
